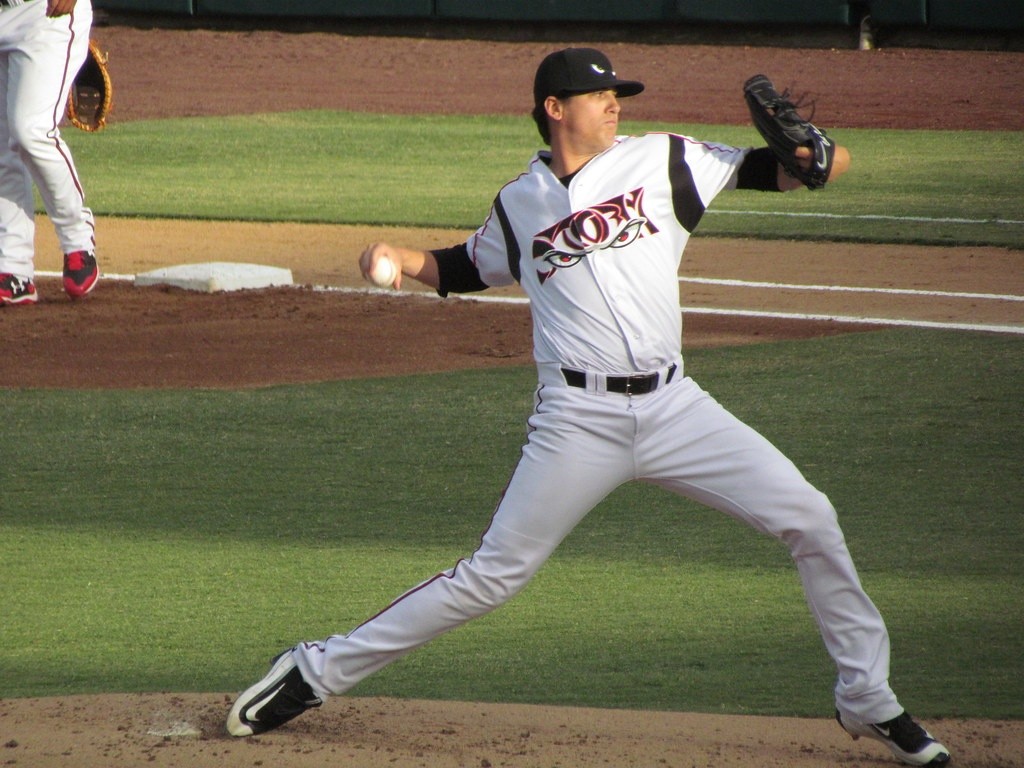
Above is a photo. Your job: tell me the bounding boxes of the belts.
[0,0,31,5]
[561,365,676,397]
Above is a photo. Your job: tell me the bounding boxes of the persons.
[0,0,99,306]
[226,47,951,768]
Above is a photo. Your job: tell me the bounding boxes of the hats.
[535,47,644,98]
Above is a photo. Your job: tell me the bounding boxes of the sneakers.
[837,705,950,768]
[63,245,98,296]
[0,273,38,306]
[225,647,322,737]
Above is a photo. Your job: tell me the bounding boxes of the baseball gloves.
[741,73,837,192]
[66,37,114,134]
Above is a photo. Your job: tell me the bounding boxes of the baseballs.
[365,255,398,289]
